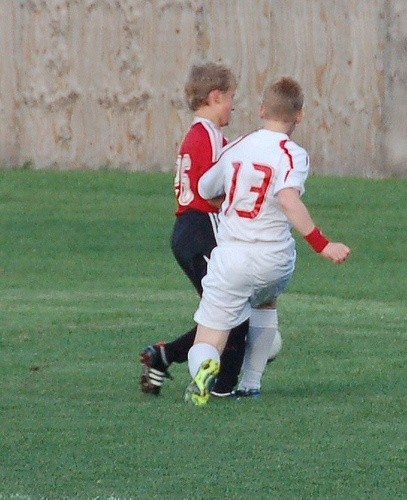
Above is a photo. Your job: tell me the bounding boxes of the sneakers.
[233,387,260,400]
[138,342,169,396]
[209,389,236,398]
[183,358,221,405]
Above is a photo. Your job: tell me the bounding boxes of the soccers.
[268,331,283,361]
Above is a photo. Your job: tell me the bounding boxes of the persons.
[137,60,252,398]
[181,75,351,408]
